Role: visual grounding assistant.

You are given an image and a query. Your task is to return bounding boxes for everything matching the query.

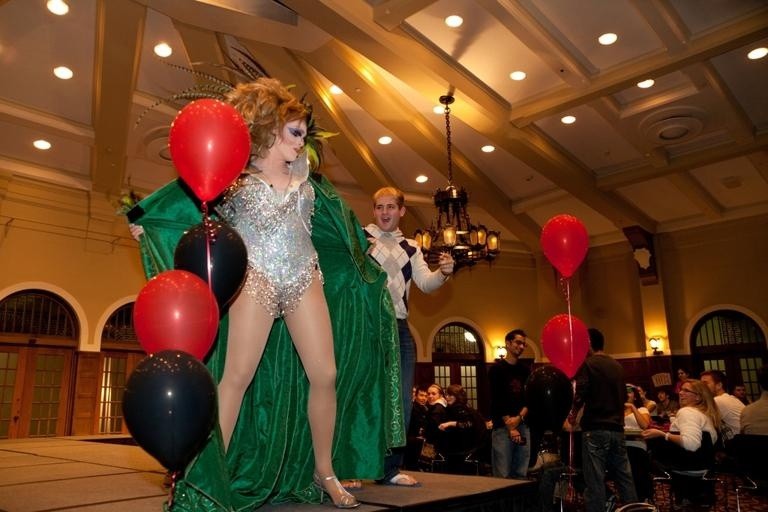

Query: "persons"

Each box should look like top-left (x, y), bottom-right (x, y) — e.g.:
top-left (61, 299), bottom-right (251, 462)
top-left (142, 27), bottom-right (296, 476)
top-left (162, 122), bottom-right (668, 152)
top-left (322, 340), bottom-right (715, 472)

top-left (358, 182), bottom-right (457, 487)
top-left (124, 75), bottom-right (409, 512)
top-left (412, 383), bottom-right (474, 471)
top-left (623, 367), bottom-right (768, 486)
top-left (567, 327), bottom-right (638, 511)
top-left (476, 327), bottom-right (534, 480)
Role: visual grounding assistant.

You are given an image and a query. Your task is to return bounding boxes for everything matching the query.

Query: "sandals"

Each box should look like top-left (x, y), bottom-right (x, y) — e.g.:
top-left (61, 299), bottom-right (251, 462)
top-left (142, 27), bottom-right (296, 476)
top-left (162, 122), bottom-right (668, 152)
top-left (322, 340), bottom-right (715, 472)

top-left (390, 473), bottom-right (421, 487)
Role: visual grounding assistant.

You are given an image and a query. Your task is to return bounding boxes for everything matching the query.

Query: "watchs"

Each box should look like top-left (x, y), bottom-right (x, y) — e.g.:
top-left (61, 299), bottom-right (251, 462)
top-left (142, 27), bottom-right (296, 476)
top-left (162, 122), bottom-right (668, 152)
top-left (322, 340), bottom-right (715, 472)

top-left (517, 412), bottom-right (526, 424)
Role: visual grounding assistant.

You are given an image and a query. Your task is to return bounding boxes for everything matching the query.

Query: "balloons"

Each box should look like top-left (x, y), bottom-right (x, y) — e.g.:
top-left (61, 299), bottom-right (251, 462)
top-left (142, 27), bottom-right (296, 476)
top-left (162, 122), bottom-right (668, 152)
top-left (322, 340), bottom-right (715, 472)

top-left (172, 219), bottom-right (248, 310)
top-left (120, 351), bottom-right (221, 476)
top-left (132, 268), bottom-right (222, 362)
top-left (540, 314), bottom-right (590, 382)
top-left (539, 213), bottom-right (591, 283)
top-left (168, 97), bottom-right (251, 202)
top-left (524, 368), bottom-right (573, 433)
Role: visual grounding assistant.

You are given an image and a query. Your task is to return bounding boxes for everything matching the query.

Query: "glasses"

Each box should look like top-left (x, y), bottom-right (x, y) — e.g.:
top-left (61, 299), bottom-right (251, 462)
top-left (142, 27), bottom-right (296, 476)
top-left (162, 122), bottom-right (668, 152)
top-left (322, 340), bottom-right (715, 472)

top-left (680, 387), bottom-right (698, 395)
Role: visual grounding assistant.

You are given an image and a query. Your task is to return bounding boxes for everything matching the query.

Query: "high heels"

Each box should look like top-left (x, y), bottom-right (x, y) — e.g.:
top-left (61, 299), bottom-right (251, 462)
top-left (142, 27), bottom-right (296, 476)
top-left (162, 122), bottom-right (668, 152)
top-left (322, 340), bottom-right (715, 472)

top-left (313, 472), bottom-right (361, 509)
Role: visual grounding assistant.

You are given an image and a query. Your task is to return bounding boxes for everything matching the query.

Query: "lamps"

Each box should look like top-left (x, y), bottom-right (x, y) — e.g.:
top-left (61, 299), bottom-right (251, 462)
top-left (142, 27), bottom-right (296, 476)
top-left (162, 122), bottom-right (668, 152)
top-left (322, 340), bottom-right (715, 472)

top-left (648, 337), bottom-right (664, 356)
top-left (496, 345), bottom-right (506, 358)
top-left (415, 93), bottom-right (500, 270)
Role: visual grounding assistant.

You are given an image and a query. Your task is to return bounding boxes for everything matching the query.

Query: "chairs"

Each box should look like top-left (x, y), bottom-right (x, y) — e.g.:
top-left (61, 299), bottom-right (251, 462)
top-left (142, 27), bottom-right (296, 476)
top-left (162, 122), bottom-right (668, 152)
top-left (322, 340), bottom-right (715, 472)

top-left (399, 430), bottom-right (767, 511)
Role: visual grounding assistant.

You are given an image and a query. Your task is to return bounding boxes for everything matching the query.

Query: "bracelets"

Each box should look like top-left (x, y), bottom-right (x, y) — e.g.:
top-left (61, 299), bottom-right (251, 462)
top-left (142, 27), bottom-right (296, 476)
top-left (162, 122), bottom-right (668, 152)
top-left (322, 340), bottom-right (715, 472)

top-left (569, 410), bottom-right (577, 418)
